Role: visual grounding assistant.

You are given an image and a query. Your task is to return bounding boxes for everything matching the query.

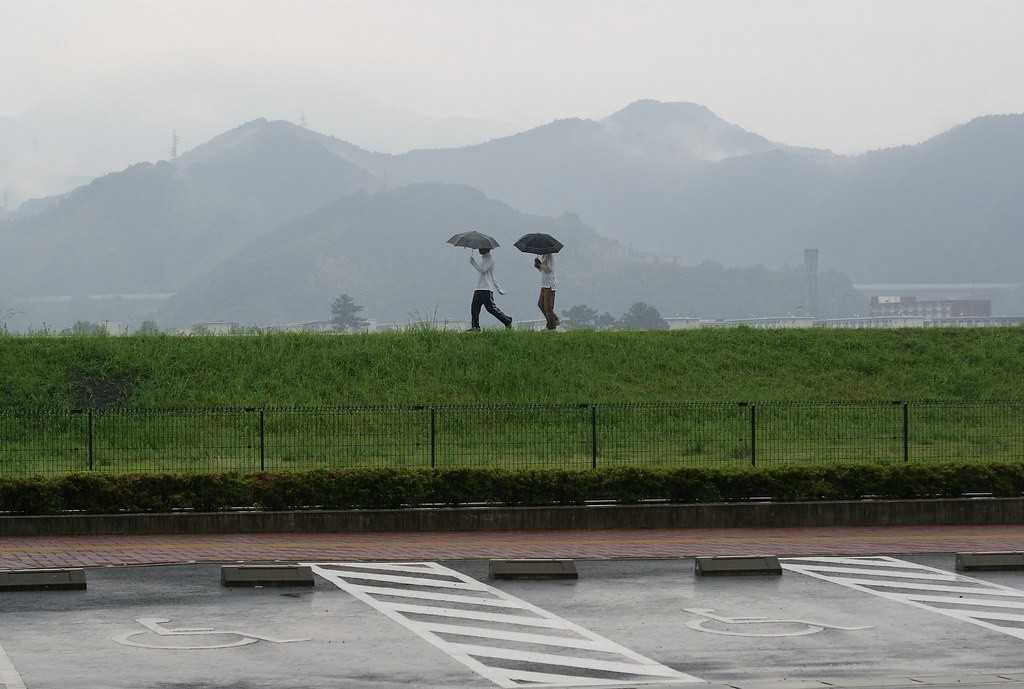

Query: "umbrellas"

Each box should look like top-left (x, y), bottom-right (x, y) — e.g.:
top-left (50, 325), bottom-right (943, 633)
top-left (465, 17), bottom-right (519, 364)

top-left (446, 231), bottom-right (500, 257)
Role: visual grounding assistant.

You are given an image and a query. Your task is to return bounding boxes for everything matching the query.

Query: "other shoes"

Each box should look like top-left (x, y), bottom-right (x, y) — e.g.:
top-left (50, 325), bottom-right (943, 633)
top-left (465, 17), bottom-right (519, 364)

top-left (466, 327), bottom-right (481, 332)
top-left (546, 318), bottom-right (561, 328)
top-left (506, 317), bottom-right (512, 330)
top-left (542, 328), bottom-right (557, 332)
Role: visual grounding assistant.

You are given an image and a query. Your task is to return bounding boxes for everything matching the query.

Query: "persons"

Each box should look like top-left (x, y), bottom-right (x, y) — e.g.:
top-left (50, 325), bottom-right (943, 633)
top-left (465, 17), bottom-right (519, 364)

top-left (534, 253), bottom-right (560, 331)
top-left (467, 248), bottom-right (513, 332)
top-left (513, 232), bottom-right (564, 256)
